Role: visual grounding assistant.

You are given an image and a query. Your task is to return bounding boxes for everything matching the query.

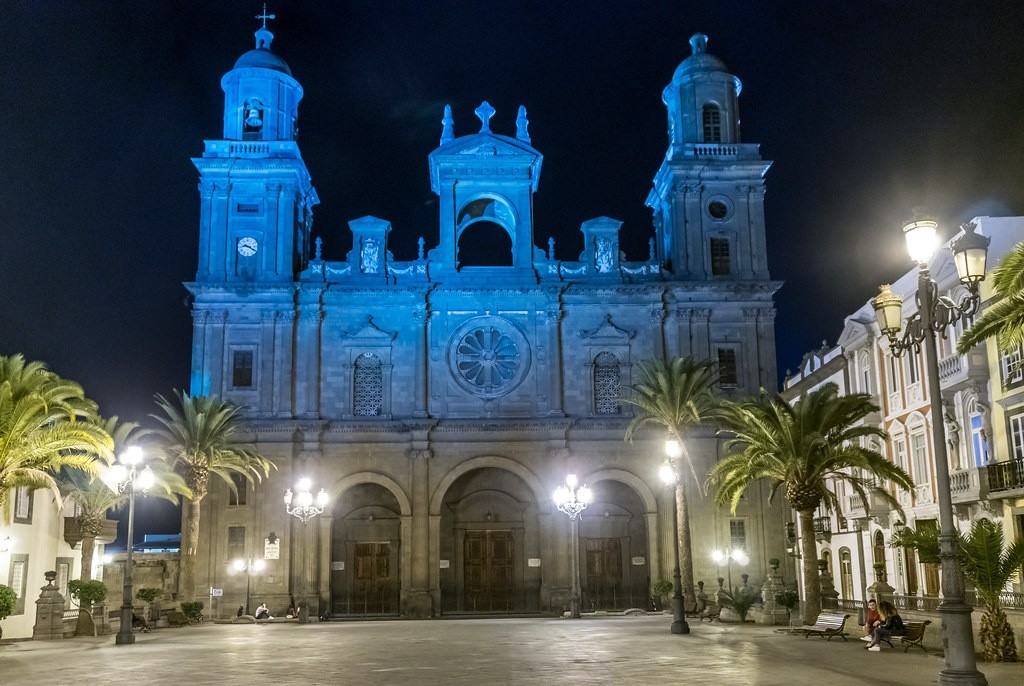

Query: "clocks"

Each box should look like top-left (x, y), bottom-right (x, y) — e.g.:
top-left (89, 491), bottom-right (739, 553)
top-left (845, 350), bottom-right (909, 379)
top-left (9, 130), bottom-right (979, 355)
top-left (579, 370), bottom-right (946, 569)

top-left (238, 237), bottom-right (258, 257)
top-left (787, 544), bottom-right (802, 559)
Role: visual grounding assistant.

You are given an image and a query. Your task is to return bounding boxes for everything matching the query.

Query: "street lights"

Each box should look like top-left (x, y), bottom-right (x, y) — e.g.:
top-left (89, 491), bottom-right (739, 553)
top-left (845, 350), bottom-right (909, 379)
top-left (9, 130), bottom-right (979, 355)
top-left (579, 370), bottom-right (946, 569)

top-left (283, 477), bottom-right (329, 621)
top-left (712, 548), bottom-right (750, 596)
top-left (872, 203), bottom-right (992, 686)
top-left (111, 446), bottom-right (156, 642)
top-left (553, 473), bottom-right (592, 618)
top-left (234, 557), bottom-right (266, 615)
top-left (658, 427), bottom-right (691, 633)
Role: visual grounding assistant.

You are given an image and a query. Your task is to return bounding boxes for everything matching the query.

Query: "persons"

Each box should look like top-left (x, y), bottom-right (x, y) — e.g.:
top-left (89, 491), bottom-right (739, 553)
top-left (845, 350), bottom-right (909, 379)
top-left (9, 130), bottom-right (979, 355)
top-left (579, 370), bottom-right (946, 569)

top-left (863, 599), bottom-right (881, 648)
top-left (976, 402), bottom-right (993, 462)
top-left (944, 414), bottom-right (961, 470)
top-left (295, 603), bottom-right (300, 615)
top-left (319, 608), bottom-right (330, 622)
top-left (255, 603), bottom-right (274, 619)
top-left (285, 603), bottom-right (296, 619)
top-left (860, 601), bottom-right (905, 651)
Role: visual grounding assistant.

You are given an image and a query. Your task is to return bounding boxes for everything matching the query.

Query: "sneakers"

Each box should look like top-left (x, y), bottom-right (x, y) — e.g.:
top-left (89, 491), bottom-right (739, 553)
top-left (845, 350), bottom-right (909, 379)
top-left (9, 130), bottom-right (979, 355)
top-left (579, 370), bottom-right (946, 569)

top-left (868, 646), bottom-right (880, 651)
top-left (859, 635), bottom-right (872, 642)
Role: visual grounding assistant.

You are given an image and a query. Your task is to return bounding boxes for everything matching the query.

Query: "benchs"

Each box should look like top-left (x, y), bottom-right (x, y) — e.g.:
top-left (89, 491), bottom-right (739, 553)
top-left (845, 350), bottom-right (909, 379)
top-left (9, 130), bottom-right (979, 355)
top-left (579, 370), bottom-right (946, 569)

top-left (166, 612), bottom-right (194, 628)
top-left (132, 611), bottom-right (152, 633)
top-left (801, 612), bottom-right (851, 641)
top-left (698, 604), bottom-right (723, 623)
top-left (684, 602), bottom-right (698, 618)
top-left (864, 619), bottom-right (931, 653)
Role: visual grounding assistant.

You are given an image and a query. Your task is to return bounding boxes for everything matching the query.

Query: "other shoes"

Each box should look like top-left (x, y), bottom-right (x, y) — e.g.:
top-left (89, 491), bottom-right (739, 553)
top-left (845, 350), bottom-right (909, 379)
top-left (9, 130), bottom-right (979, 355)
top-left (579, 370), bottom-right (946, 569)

top-left (864, 644), bottom-right (869, 649)
top-left (268, 616), bottom-right (275, 619)
